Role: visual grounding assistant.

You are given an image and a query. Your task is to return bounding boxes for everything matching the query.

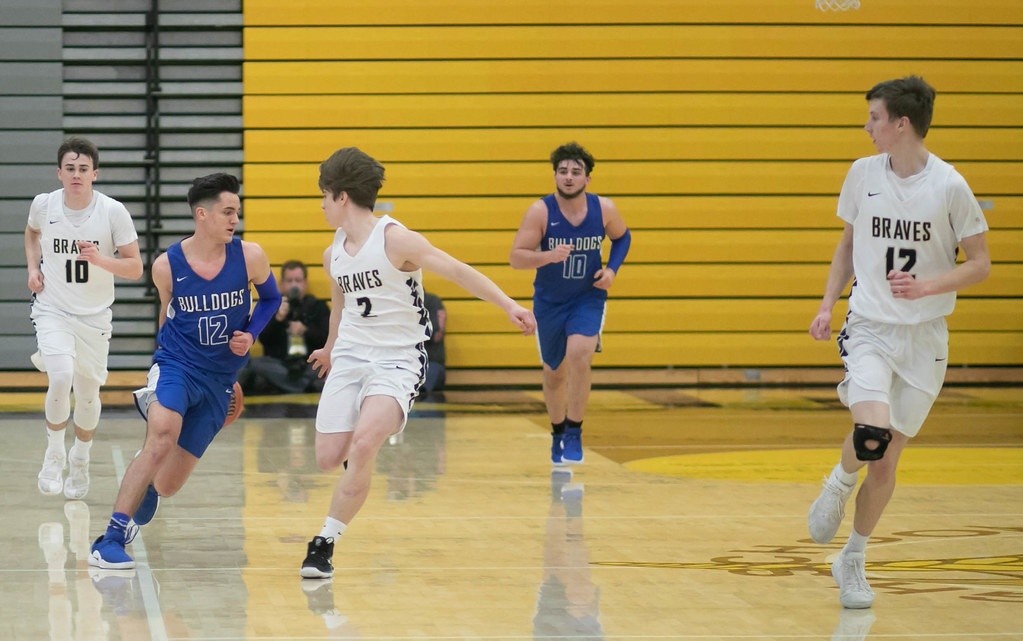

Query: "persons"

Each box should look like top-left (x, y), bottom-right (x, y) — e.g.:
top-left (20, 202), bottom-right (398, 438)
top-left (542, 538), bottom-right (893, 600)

top-left (25, 139), bottom-right (143, 500)
top-left (236, 261), bottom-right (331, 395)
top-left (87, 174), bottom-right (282, 569)
top-left (510, 141), bottom-right (631, 466)
top-left (805, 76), bottom-right (990, 609)
top-left (416, 287), bottom-right (448, 404)
top-left (299, 148), bottom-right (537, 578)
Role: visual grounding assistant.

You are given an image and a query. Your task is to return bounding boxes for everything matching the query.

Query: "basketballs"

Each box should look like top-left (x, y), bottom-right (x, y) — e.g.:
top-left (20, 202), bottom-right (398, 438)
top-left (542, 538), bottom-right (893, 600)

top-left (223, 381), bottom-right (244, 428)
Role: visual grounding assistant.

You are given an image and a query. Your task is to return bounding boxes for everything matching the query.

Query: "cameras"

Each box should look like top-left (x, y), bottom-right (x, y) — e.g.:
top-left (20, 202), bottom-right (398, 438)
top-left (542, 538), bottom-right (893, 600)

top-left (283, 287), bottom-right (303, 321)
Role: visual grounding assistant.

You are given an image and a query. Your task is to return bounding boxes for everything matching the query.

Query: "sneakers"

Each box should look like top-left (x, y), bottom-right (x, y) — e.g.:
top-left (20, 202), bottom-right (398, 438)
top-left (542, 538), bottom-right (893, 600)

top-left (38, 451), bottom-right (67, 495)
top-left (39, 522), bottom-right (68, 564)
top-left (832, 608), bottom-right (877, 641)
top-left (808, 467), bottom-right (857, 544)
top-left (64, 500), bottom-right (90, 553)
top-left (64, 446), bottom-right (90, 499)
top-left (831, 544), bottom-right (875, 609)
top-left (87, 523), bottom-right (140, 569)
top-left (87, 566), bottom-right (137, 616)
top-left (300, 576), bottom-right (335, 615)
top-left (551, 468), bottom-right (573, 503)
top-left (561, 483), bottom-right (584, 514)
top-left (560, 427), bottom-right (584, 463)
top-left (344, 459), bottom-right (348, 470)
top-left (550, 431), bottom-right (570, 466)
top-left (131, 571), bottom-right (160, 612)
top-left (133, 450), bottom-right (161, 525)
top-left (301, 536), bottom-right (334, 577)
